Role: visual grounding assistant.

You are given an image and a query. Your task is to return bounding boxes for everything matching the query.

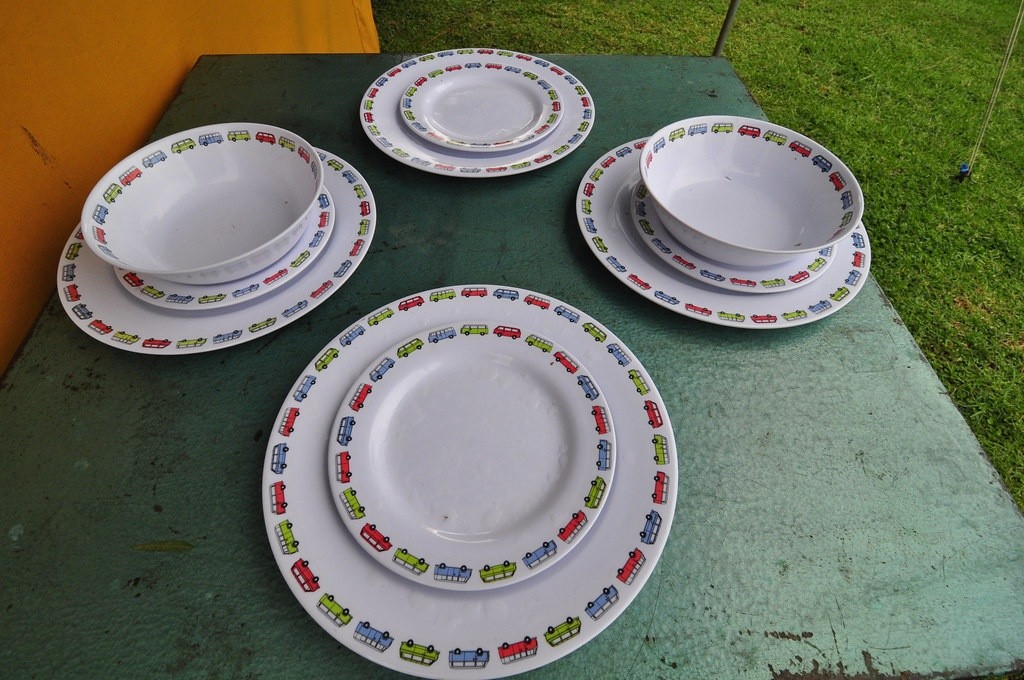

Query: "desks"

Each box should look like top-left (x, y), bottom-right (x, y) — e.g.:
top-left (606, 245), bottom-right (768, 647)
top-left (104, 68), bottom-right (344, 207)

top-left (0, 50), bottom-right (1024, 680)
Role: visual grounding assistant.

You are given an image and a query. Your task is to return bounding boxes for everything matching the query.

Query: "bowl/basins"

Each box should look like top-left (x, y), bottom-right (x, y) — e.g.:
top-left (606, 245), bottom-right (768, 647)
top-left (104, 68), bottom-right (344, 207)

top-left (640, 114), bottom-right (864, 266)
top-left (82, 123), bottom-right (323, 286)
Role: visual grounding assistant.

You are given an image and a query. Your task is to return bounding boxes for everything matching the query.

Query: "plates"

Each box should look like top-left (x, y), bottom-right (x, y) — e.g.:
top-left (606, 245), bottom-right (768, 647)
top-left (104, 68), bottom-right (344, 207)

top-left (57, 147), bottom-right (377, 355)
top-left (631, 179), bottom-right (836, 293)
top-left (575, 136), bottom-right (871, 330)
top-left (326, 320), bottom-right (617, 591)
top-left (261, 284), bottom-right (679, 680)
top-left (112, 186), bottom-right (336, 310)
top-left (360, 48), bottom-right (595, 178)
top-left (399, 62), bottom-right (564, 153)
